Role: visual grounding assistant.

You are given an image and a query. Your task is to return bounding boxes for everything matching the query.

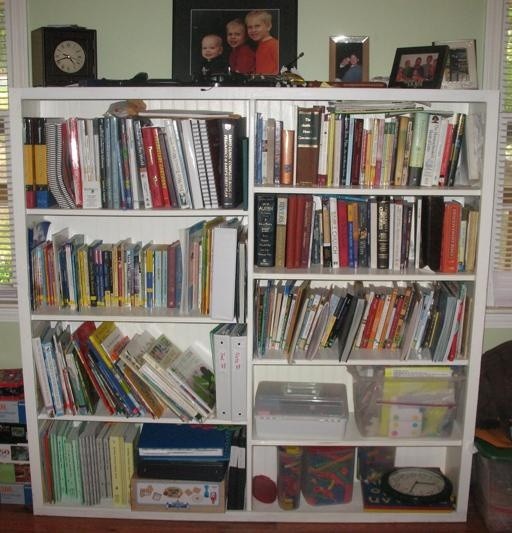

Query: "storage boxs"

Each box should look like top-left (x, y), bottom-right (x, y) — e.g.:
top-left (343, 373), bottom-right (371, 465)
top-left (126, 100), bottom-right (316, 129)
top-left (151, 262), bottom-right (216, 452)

top-left (351, 376), bottom-right (466, 439)
top-left (474, 427), bottom-right (512, 533)
top-left (255, 381), bottom-right (349, 441)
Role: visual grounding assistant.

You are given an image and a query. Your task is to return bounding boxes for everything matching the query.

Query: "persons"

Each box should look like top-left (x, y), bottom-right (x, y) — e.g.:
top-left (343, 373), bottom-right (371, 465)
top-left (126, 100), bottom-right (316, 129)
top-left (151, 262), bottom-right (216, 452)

top-left (189, 9), bottom-right (445, 88)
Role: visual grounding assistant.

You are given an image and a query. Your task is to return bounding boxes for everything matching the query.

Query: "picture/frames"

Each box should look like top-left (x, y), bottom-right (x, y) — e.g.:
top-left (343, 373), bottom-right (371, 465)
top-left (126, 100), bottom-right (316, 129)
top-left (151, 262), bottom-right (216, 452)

top-left (388, 45), bottom-right (449, 88)
top-left (329, 37), bottom-right (370, 83)
top-left (172, 1), bottom-right (298, 87)
top-left (434, 39), bottom-right (479, 89)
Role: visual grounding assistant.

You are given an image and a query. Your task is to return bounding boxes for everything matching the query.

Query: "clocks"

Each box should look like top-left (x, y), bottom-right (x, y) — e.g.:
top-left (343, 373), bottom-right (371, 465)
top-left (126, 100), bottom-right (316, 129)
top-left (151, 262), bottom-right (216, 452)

top-left (31, 24), bottom-right (97, 87)
top-left (384, 467), bottom-right (453, 504)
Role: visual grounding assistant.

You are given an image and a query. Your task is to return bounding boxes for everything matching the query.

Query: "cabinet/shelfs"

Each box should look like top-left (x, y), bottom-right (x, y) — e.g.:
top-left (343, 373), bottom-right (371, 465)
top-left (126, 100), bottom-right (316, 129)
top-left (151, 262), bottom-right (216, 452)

top-left (8, 87), bottom-right (503, 523)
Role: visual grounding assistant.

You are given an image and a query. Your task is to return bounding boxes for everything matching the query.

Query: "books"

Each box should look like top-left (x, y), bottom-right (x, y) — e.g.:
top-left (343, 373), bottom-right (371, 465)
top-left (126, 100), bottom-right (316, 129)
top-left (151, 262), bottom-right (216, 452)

top-left (255, 101), bottom-right (481, 188)
top-left (31, 320), bottom-right (216, 423)
top-left (27, 217), bottom-right (246, 324)
top-left (255, 280), bottom-right (473, 363)
top-left (136, 422), bottom-right (229, 481)
top-left (24, 109), bottom-right (246, 210)
top-left (227, 445), bottom-right (245, 510)
top-left (256, 193), bottom-right (480, 273)
top-left (38, 421), bottom-right (140, 508)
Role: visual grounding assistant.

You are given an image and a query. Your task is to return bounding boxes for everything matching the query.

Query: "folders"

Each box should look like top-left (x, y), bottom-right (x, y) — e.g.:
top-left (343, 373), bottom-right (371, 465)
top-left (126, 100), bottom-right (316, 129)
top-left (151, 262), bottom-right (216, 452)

top-left (213, 326), bottom-right (247, 423)
